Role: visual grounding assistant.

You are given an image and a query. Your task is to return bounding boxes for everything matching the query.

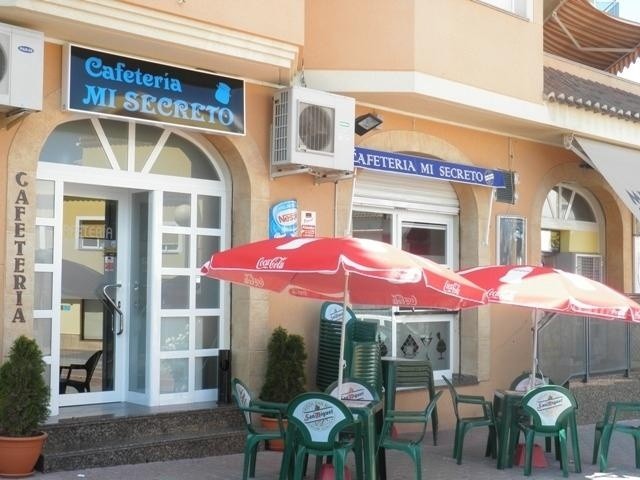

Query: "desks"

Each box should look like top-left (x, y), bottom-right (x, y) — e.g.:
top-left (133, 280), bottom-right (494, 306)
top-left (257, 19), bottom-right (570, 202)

top-left (300, 395), bottom-right (389, 480)
top-left (482, 385), bottom-right (567, 471)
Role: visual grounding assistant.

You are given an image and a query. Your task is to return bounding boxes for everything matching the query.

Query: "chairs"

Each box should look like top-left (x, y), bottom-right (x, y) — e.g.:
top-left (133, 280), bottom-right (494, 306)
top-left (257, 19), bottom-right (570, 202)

top-left (326, 370), bottom-right (381, 410)
top-left (275, 391), bottom-right (363, 478)
top-left (374, 390), bottom-right (448, 480)
top-left (59, 349), bottom-right (108, 397)
top-left (312, 297), bottom-right (444, 445)
top-left (435, 368), bottom-right (505, 467)
top-left (507, 384), bottom-right (587, 480)
top-left (507, 369), bottom-right (560, 451)
top-left (589, 395), bottom-right (640, 474)
top-left (231, 372), bottom-right (298, 479)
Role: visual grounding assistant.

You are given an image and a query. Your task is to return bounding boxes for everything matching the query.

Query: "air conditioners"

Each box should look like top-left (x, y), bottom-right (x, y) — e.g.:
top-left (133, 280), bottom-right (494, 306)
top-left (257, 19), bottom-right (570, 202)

top-left (0, 21), bottom-right (46, 116)
top-left (270, 82), bottom-right (359, 182)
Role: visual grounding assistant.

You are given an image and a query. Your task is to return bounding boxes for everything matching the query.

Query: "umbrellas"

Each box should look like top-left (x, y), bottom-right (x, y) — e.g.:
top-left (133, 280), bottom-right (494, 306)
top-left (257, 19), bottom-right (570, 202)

top-left (450, 263), bottom-right (640, 393)
top-left (197, 235), bottom-right (491, 405)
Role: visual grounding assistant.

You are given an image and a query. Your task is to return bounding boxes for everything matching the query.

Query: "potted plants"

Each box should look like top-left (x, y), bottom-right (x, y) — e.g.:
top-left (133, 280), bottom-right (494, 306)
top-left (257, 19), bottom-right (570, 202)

top-left (254, 321), bottom-right (310, 452)
top-left (0, 333), bottom-right (52, 477)
